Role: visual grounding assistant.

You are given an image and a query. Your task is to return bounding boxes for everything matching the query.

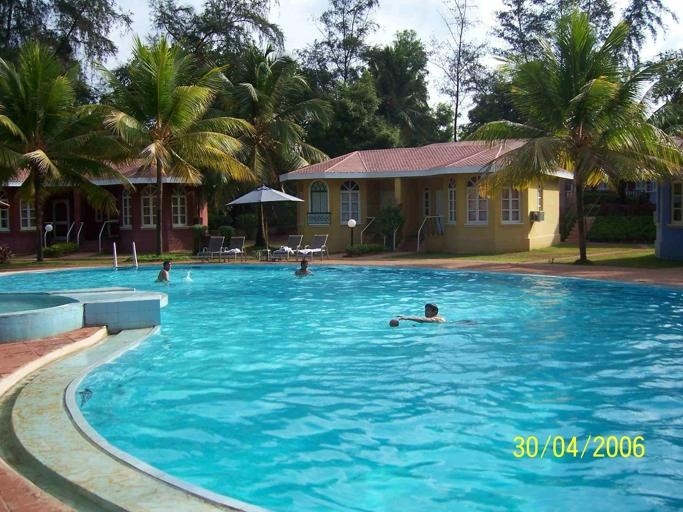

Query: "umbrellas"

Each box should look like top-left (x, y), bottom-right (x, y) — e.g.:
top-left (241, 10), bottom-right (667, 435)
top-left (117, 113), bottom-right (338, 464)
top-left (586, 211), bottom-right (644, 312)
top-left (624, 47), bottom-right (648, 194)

top-left (225, 183), bottom-right (305, 250)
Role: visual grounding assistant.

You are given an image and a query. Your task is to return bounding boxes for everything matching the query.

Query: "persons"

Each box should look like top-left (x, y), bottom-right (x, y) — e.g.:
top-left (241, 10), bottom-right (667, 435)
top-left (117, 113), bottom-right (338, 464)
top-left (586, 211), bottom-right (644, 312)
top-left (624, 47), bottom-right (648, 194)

top-left (293, 259), bottom-right (313, 276)
top-left (154, 260), bottom-right (172, 283)
top-left (395, 302), bottom-right (446, 324)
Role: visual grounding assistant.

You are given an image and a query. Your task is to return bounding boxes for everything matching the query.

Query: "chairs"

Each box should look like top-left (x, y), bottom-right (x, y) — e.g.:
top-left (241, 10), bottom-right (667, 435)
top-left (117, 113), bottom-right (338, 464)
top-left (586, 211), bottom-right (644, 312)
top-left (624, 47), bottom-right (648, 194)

top-left (195, 234), bottom-right (330, 262)
